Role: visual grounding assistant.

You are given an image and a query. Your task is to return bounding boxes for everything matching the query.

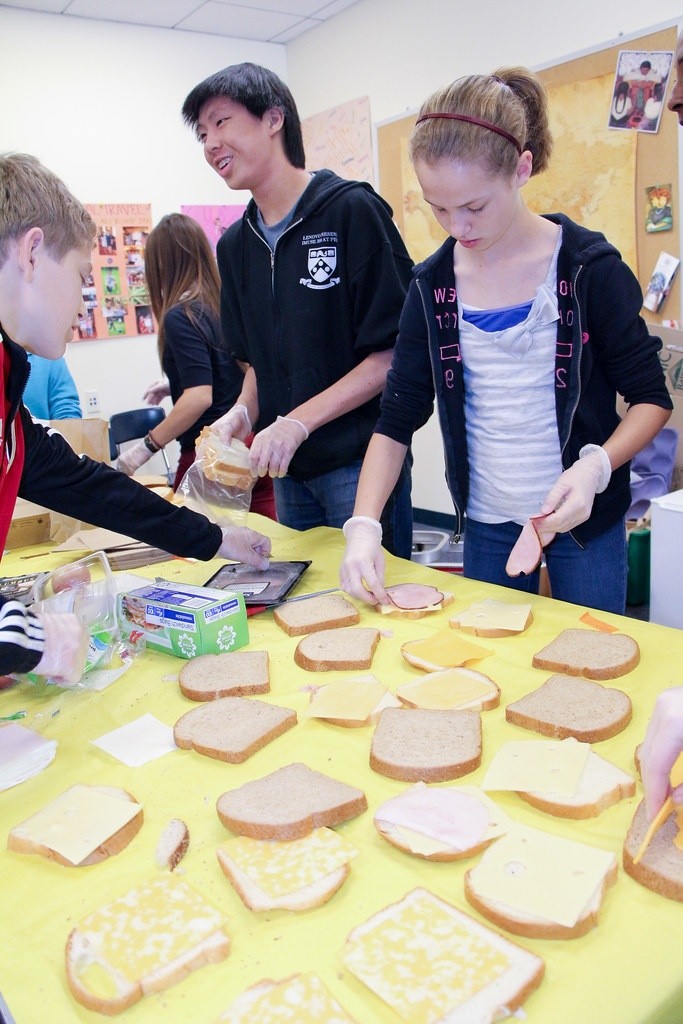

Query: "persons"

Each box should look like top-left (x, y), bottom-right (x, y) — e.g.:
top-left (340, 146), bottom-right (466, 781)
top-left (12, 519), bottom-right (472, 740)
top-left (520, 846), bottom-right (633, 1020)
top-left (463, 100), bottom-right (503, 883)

top-left (180, 62), bottom-right (416, 560)
top-left (21, 350), bottom-right (82, 420)
top-left (0, 150), bottom-right (271, 688)
top-left (115, 214), bottom-right (275, 521)
top-left (636, 30), bottom-right (683, 827)
top-left (341, 67), bottom-right (674, 616)
top-left (77, 227), bottom-right (154, 337)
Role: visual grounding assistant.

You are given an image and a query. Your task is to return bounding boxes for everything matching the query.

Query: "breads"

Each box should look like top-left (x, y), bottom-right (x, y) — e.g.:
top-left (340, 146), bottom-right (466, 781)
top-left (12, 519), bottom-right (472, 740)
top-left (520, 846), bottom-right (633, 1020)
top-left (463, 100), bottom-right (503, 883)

top-left (174, 697), bottom-right (298, 763)
top-left (274, 594), bottom-right (360, 636)
top-left (373, 786), bottom-right (497, 861)
top-left (8, 784), bottom-right (142, 867)
top-left (347, 891), bottom-right (546, 1024)
top-left (622, 785), bottom-right (683, 902)
top-left (533, 628), bottom-right (641, 679)
top-left (374, 588), bottom-right (452, 619)
top-left (219, 974), bottom-right (358, 1024)
top-left (154, 818), bottom-right (190, 871)
top-left (396, 666), bottom-right (500, 713)
top-left (294, 627), bottom-right (380, 671)
top-left (510, 738), bottom-right (637, 819)
top-left (402, 640), bottom-right (465, 671)
top-left (466, 824), bottom-right (618, 940)
top-left (194, 426), bottom-right (254, 489)
top-left (150, 486), bottom-right (174, 501)
top-left (506, 672), bottom-right (633, 743)
top-left (66, 884), bottom-right (231, 1015)
top-left (180, 652), bottom-right (269, 701)
top-left (311, 675), bottom-right (400, 729)
top-left (450, 599), bottom-right (532, 637)
top-left (216, 827), bottom-right (350, 914)
top-left (370, 707), bottom-right (483, 782)
top-left (217, 765), bottom-right (367, 841)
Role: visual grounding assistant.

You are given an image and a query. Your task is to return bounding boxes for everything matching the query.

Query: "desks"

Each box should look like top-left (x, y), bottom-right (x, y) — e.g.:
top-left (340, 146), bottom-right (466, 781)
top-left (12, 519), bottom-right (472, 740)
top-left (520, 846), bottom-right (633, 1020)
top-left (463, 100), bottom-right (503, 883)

top-left (1, 495), bottom-right (683, 1024)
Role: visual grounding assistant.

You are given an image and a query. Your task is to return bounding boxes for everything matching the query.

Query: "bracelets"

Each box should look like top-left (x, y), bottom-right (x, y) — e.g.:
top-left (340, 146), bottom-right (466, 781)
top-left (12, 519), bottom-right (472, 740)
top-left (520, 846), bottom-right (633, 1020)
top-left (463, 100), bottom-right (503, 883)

top-left (148, 430), bottom-right (165, 449)
top-left (144, 434), bottom-right (159, 453)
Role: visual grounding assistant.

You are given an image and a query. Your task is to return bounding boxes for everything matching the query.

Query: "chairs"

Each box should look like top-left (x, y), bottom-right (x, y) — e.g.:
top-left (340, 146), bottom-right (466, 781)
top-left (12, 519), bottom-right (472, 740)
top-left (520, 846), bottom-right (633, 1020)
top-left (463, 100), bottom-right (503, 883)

top-left (110, 407), bottom-right (174, 487)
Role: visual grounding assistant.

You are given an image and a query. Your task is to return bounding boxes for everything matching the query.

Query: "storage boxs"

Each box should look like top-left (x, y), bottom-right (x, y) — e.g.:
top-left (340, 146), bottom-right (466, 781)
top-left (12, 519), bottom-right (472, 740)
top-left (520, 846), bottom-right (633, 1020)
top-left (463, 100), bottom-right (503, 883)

top-left (116, 576), bottom-right (250, 661)
